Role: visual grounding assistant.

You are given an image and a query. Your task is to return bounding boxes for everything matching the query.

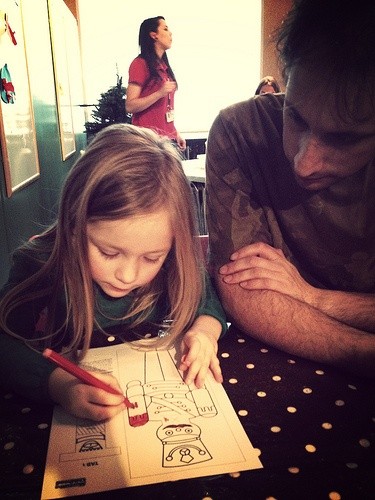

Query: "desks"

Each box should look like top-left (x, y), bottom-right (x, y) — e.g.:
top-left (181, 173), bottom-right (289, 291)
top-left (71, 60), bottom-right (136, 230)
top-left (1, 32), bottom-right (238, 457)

top-left (0, 319), bottom-right (375, 500)
top-left (183, 154), bottom-right (210, 261)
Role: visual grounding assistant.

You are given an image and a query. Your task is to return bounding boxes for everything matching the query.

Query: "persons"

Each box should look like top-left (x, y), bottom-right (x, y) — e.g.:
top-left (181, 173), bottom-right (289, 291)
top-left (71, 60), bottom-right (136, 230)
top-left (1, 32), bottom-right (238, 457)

top-left (255, 77), bottom-right (282, 99)
top-left (0, 123), bottom-right (226, 421)
top-left (206, 0), bottom-right (375, 362)
top-left (125, 17), bottom-right (186, 153)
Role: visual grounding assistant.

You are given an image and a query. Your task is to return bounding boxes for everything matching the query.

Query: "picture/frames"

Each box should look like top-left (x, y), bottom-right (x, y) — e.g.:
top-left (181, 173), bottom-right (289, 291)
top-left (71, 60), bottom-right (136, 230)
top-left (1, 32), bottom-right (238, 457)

top-left (0, 0), bottom-right (43, 201)
top-left (46, 0), bottom-right (77, 162)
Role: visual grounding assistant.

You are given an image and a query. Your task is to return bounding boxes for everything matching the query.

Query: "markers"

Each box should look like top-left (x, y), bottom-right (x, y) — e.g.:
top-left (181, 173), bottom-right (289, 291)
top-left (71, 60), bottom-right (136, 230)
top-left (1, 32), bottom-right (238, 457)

top-left (43, 347), bottom-right (136, 410)
